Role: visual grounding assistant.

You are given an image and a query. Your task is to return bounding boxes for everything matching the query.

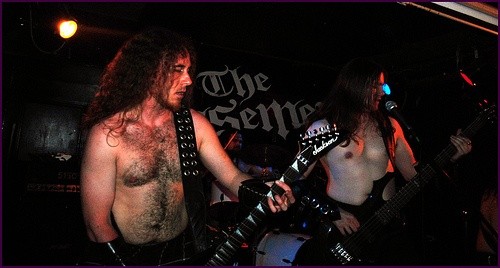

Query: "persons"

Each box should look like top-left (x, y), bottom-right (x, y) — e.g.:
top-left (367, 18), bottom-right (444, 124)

top-left (210, 128), bottom-right (275, 202)
top-left (288, 56), bottom-right (473, 237)
top-left (75, 30), bottom-right (295, 267)
top-left (475, 165), bottom-right (500, 268)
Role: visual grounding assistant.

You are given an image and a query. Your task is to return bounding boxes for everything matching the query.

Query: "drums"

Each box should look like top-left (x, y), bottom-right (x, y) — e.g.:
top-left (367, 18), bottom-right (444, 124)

top-left (253, 227), bottom-right (313, 267)
top-left (207, 176), bottom-right (249, 228)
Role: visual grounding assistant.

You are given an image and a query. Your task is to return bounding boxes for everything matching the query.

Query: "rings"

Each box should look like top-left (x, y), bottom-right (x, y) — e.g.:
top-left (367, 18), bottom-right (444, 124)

top-left (467, 142), bottom-right (471, 145)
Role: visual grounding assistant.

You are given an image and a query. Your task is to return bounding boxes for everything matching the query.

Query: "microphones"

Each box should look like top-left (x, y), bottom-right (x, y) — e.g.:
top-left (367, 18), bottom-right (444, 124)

top-left (385, 100), bottom-right (410, 130)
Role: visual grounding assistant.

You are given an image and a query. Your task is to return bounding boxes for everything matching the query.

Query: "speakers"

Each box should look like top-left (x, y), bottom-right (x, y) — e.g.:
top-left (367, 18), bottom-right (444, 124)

top-left (476, 189), bottom-right (497, 255)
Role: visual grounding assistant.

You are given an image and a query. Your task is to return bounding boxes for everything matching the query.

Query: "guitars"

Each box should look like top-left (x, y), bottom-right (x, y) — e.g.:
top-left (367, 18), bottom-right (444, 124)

top-left (204, 115), bottom-right (344, 266)
top-left (291, 94), bottom-right (499, 267)
